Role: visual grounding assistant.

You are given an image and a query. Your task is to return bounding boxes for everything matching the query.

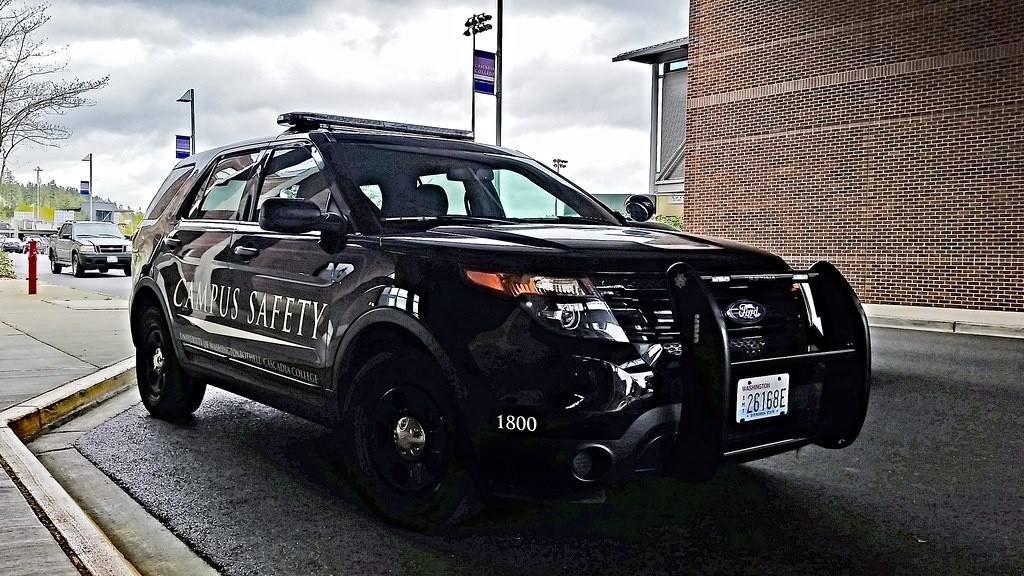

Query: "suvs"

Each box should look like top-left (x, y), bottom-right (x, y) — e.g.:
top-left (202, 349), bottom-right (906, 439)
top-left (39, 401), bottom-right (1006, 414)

top-left (48, 220), bottom-right (133, 278)
top-left (120, 111), bottom-right (874, 534)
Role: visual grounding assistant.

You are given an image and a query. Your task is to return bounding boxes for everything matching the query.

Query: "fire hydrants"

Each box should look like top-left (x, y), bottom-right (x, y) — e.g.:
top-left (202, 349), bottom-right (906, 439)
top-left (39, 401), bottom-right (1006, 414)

top-left (23, 238), bottom-right (39, 296)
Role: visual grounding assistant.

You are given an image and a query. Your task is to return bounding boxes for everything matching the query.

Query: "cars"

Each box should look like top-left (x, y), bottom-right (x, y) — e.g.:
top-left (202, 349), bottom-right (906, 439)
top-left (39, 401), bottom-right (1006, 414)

top-left (2, 237), bottom-right (22, 254)
top-left (19, 235), bottom-right (47, 254)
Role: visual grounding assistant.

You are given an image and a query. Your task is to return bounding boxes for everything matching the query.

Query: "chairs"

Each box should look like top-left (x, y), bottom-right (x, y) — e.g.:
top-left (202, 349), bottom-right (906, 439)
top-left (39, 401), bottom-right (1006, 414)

top-left (293, 168), bottom-right (365, 213)
top-left (406, 183), bottom-right (450, 218)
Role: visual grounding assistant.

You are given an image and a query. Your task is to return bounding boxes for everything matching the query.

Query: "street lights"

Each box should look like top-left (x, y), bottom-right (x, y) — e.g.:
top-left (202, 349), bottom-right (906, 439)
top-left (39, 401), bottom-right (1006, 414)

top-left (36, 169), bottom-right (45, 219)
top-left (552, 159), bottom-right (569, 215)
top-left (462, 12), bottom-right (493, 140)
top-left (81, 152), bottom-right (92, 221)
top-left (175, 88), bottom-right (196, 155)
